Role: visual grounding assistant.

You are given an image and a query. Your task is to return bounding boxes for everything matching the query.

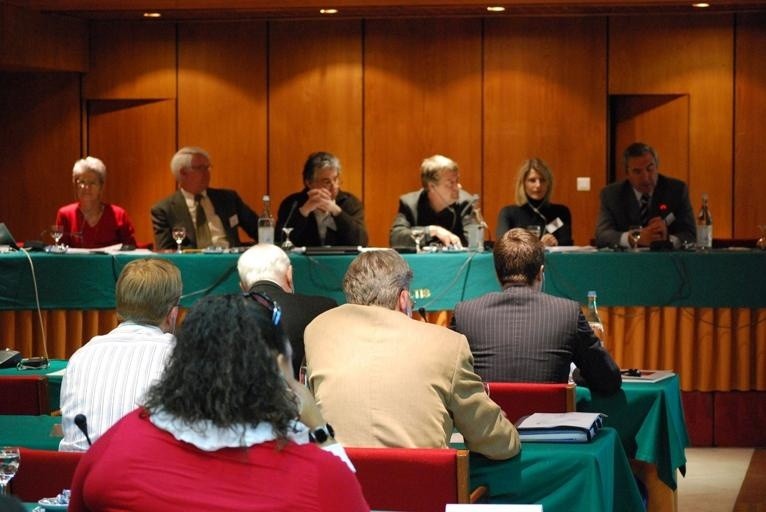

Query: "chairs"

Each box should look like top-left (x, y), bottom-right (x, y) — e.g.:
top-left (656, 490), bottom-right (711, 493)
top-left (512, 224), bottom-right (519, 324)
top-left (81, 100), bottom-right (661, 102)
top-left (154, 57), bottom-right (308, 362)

top-left (5, 447), bottom-right (86, 502)
top-left (0, 376), bottom-right (50, 415)
top-left (481, 383), bottom-right (576, 425)
top-left (344, 447), bottom-right (469, 512)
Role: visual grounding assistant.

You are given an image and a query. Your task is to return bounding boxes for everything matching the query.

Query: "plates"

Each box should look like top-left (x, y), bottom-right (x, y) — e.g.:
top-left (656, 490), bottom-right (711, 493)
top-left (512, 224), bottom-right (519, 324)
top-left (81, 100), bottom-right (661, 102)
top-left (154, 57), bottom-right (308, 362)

top-left (37, 497), bottom-right (70, 511)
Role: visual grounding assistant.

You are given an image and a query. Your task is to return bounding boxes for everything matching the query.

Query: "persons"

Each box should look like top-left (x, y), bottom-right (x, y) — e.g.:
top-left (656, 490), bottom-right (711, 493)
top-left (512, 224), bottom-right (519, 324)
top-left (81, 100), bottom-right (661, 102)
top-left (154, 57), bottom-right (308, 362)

top-left (57, 256), bottom-right (184, 454)
top-left (448, 226), bottom-right (623, 398)
top-left (495, 157), bottom-right (574, 247)
top-left (69, 290), bottom-right (372, 512)
top-left (303, 248), bottom-right (522, 463)
top-left (593, 142), bottom-right (696, 252)
top-left (56, 154), bottom-right (139, 249)
top-left (237, 242), bottom-right (340, 383)
top-left (275, 151), bottom-right (369, 248)
top-left (389, 153), bottom-right (476, 253)
top-left (150, 145), bottom-right (260, 251)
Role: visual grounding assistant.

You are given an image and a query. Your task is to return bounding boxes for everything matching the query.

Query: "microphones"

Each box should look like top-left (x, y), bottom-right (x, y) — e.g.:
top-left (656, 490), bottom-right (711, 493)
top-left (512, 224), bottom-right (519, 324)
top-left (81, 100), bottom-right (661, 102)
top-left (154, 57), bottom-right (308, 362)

top-left (651, 188), bottom-right (673, 251)
top-left (75, 413), bottom-right (93, 446)
top-left (262, 195), bottom-right (273, 218)
top-left (418, 306), bottom-right (427, 322)
top-left (461, 193), bottom-right (481, 218)
top-left (278, 201), bottom-right (298, 247)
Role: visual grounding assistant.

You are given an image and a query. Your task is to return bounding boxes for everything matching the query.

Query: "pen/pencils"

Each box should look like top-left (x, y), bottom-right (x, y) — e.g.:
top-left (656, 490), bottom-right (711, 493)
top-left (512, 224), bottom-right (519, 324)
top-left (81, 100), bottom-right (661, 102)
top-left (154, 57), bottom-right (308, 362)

top-left (184, 249), bottom-right (204, 253)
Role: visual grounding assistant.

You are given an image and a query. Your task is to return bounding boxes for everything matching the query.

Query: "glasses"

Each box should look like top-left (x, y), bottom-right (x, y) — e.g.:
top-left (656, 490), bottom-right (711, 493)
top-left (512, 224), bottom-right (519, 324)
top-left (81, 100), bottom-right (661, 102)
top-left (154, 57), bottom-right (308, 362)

top-left (242, 290), bottom-right (283, 326)
top-left (76, 179), bottom-right (103, 188)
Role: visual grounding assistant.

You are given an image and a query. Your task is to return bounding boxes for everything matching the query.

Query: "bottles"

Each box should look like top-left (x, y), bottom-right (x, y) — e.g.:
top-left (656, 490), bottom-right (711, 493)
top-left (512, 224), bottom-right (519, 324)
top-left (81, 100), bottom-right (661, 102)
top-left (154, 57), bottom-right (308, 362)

top-left (468, 198), bottom-right (485, 255)
top-left (585, 289), bottom-right (605, 348)
top-left (697, 192), bottom-right (713, 252)
top-left (256, 193), bottom-right (276, 247)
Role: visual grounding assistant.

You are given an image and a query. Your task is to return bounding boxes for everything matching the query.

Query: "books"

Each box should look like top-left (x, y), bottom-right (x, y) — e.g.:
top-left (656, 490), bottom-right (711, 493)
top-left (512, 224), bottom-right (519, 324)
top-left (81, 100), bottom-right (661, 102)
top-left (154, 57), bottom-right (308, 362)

top-left (514, 414), bottom-right (605, 443)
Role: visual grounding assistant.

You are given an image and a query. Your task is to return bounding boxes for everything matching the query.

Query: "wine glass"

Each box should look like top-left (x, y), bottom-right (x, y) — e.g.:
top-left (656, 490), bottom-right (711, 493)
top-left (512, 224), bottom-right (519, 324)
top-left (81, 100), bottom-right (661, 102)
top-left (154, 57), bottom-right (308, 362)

top-left (0, 446), bottom-right (20, 495)
top-left (627, 225), bottom-right (643, 253)
top-left (410, 225), bottom-right (426, 254)
top-left (48, 225), bottom-right (63, 255)
top-left (172, 226), bottom-right (186, 254)
top-left (280, 227), bottom-right (296, 253)
top-left (754, 224), bottom-right (766, 251)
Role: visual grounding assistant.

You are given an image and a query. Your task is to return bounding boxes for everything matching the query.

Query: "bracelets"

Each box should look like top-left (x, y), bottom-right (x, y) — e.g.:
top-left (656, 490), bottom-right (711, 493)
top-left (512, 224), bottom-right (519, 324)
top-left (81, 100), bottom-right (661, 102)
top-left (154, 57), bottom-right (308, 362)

top-left (308, 422), bottom-right (335, 444)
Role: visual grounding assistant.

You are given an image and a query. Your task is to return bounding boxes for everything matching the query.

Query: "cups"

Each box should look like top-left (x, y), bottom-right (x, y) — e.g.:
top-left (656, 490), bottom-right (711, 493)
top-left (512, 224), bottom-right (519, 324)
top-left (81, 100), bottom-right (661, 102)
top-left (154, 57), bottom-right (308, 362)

top-left (528, 225), bottom-right (541, 238)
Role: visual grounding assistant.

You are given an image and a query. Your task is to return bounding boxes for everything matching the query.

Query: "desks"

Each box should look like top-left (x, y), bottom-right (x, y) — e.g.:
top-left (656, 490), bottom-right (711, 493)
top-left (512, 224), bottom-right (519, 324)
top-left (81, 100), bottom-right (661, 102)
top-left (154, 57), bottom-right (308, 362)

top-left (0, 358), bottom-right (688, 512)
top-left (0, 415), bottom-right (648, 512)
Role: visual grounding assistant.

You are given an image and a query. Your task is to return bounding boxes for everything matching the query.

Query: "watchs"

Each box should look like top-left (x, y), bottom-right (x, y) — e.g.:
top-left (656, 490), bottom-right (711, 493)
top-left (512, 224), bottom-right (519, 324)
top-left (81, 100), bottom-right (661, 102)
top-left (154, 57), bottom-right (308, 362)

top-left (631, 230), bottom-right (640, 243)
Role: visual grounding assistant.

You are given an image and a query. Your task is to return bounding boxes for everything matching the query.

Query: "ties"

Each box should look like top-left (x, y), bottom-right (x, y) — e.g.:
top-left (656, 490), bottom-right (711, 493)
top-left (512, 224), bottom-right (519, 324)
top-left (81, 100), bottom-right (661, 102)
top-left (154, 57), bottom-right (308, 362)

top-left (194, 193), bottom-right (213, 249)
top-left (190, 164), bottom-right (214, 172)
top-left (640, 192), bottom-right (652, 228)
